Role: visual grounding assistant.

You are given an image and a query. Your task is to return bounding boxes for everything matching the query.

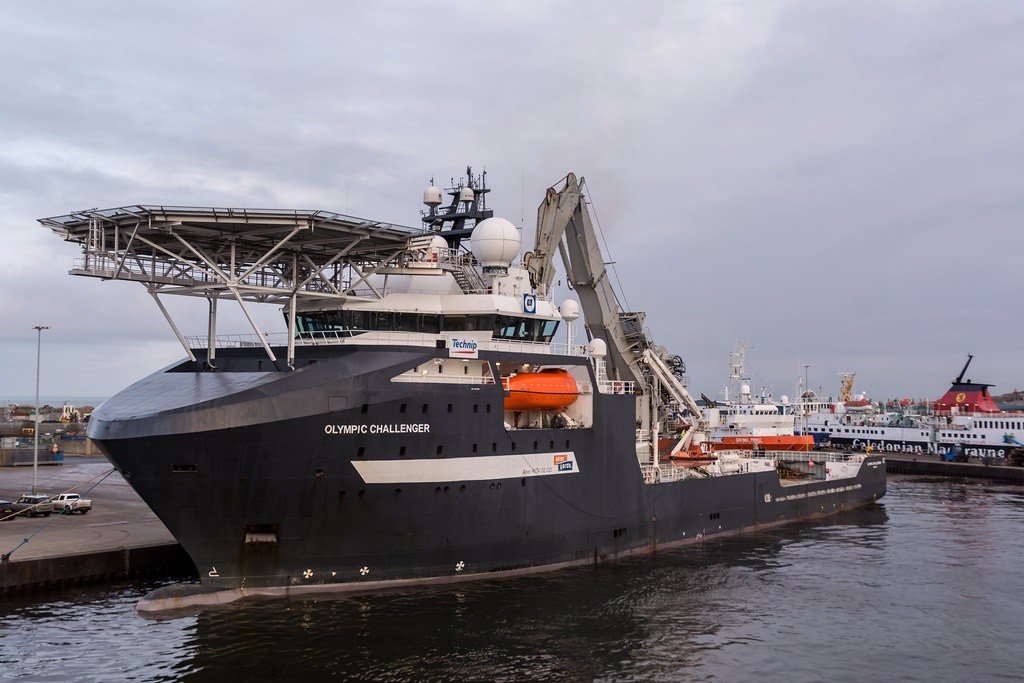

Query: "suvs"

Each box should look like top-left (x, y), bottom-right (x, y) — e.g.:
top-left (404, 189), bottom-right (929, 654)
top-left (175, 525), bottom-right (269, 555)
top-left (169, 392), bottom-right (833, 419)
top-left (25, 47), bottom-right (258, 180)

top-left (12, 493), bottom-right (54, 518)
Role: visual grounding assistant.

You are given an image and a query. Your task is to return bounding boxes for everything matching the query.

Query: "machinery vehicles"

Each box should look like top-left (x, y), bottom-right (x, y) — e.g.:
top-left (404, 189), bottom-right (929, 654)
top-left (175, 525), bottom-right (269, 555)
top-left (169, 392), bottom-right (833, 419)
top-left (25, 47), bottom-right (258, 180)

top-left (941, 445), bottom-right (972, 462)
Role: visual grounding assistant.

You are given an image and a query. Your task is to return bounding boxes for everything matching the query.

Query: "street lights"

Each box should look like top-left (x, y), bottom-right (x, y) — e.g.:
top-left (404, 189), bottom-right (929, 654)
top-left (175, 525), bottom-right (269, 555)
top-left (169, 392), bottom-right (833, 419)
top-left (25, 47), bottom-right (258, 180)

top-left (804, 366), bottom-right (810, 434)
top-left (32, 325), bottom-right (49, 495)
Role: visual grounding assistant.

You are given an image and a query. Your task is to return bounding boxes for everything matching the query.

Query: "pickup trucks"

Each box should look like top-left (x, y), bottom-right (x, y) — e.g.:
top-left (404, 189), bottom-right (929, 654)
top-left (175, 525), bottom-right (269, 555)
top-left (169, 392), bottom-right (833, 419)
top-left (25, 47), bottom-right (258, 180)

top-left (51, 493), bottom-right (92, 515)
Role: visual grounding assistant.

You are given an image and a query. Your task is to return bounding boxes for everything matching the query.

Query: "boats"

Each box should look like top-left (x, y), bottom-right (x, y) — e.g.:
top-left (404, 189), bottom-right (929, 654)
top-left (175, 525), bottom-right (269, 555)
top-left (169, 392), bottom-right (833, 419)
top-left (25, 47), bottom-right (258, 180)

top-left (36, 158), bottom-right (888, 619)
top-left (658, 339), bottom-right (1024, 455)
top-left (667, 444), bottom-right (719, 467)
top-left (501, 367), bottom-right (579, 411)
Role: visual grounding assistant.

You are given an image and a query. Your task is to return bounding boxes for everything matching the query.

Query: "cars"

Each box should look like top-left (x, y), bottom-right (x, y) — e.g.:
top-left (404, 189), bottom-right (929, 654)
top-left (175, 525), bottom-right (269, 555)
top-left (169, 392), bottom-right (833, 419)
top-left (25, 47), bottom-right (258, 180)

top-left (0, 500), bottom-right (15, 520)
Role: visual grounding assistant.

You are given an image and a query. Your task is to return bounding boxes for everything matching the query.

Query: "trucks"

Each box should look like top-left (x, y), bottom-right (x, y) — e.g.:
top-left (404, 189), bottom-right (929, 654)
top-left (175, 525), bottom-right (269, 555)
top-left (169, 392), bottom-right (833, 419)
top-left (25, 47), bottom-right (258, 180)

top-left (795, 431), bottom-right (831, 450)
